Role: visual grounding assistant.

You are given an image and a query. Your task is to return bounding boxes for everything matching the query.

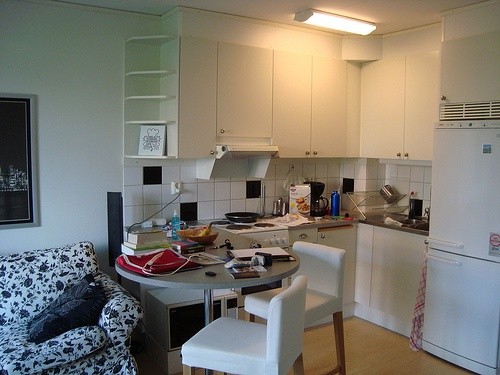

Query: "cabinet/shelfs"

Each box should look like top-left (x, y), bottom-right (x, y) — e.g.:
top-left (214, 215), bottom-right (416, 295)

top-left (239, 225), bottom-right (429, 319)
top-left (122, 7), bottom-right (443, 159)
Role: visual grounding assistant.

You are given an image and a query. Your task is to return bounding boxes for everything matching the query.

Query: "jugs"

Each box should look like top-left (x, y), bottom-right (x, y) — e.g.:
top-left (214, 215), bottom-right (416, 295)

top-left (313, 196), bottom-right (328, 211)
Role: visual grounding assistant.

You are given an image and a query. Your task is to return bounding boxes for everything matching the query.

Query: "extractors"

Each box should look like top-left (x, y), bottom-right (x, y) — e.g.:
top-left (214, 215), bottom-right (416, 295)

top-left (215, 143), bottom-right (278, 159)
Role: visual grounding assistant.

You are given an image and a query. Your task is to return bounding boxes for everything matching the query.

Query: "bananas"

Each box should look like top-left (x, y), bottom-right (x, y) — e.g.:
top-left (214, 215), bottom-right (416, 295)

top-left (195, 222), bottom-right (212, 236)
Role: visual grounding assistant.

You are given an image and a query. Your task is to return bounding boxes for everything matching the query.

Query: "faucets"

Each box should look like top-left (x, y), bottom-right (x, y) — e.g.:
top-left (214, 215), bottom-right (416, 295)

top-left (423, 207), bottom-right (430, 222)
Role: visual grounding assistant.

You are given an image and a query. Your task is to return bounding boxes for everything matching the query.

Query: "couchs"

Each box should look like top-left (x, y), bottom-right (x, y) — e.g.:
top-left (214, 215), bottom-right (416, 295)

top-left (0, 240), bottom-right (145, 375)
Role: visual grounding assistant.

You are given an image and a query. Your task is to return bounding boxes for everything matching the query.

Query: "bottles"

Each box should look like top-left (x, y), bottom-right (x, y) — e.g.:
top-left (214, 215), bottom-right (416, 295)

top-left (331, 190), bottom-right (340, 216)
top-left (273, 197), bottom-right (289, 216)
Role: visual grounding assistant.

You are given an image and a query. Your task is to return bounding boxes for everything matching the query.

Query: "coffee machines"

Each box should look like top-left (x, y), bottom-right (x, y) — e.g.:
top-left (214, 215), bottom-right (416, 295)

top-left (304, 181), bottom-right (329, 217)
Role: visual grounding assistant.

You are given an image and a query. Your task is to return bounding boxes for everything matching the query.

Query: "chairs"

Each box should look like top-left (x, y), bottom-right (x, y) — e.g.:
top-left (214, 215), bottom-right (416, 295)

top-left (181, 275), bottom-right (307, 375)
top-left (244, 241), bottom-right (347, 375)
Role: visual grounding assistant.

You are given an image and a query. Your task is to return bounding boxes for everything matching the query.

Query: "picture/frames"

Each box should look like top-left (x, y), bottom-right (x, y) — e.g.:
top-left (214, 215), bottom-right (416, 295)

top-left (0, 91), bottom-right (41, 229)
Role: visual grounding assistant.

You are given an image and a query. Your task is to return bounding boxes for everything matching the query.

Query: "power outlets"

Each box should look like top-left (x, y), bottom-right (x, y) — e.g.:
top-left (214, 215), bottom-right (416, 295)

top-left (171, 181), bottom-right (184, 195)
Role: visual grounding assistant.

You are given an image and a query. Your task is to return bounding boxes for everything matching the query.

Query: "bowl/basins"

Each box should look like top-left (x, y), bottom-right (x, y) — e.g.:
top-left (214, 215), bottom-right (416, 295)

top-left (176, 228), bottom-right (218, 245)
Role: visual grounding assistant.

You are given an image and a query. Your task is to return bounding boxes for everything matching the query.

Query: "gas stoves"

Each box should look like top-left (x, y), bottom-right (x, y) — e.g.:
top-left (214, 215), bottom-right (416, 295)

top-left (207, 220), bottom-right (289, 248)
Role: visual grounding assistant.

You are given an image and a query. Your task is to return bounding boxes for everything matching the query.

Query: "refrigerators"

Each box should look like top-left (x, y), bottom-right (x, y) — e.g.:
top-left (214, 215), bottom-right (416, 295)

top-left (421, 118), bottom-right (499, 375)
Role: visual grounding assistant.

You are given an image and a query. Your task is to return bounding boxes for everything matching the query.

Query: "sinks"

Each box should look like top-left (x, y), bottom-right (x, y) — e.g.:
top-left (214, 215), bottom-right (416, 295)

top-left (408, 223), bottom-right (429, 232)
top-left (382, 218), bottom-right (426, 227)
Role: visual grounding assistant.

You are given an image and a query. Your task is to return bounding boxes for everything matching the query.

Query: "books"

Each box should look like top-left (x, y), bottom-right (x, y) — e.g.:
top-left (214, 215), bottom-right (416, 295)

top-left (171, 238), bottom-right (205, 254)
top-left (229, 265), bottom-right (267, 279)
top-left (122, 229), bottom-right (172, 257)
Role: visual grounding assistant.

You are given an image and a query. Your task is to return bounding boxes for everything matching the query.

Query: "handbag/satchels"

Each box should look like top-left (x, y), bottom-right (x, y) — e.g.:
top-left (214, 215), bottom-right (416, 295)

top-left (117, 248), bottom-right (192, 275)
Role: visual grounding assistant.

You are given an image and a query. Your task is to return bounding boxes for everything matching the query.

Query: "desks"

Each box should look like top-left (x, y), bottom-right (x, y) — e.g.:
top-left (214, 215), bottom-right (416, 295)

top-left (114, 245), bottom-right (300, 375)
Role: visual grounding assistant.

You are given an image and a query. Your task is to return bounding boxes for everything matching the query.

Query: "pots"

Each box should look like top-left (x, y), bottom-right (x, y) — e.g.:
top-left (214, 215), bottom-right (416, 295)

top-left (225, 212), bottom-right (259, 223)
